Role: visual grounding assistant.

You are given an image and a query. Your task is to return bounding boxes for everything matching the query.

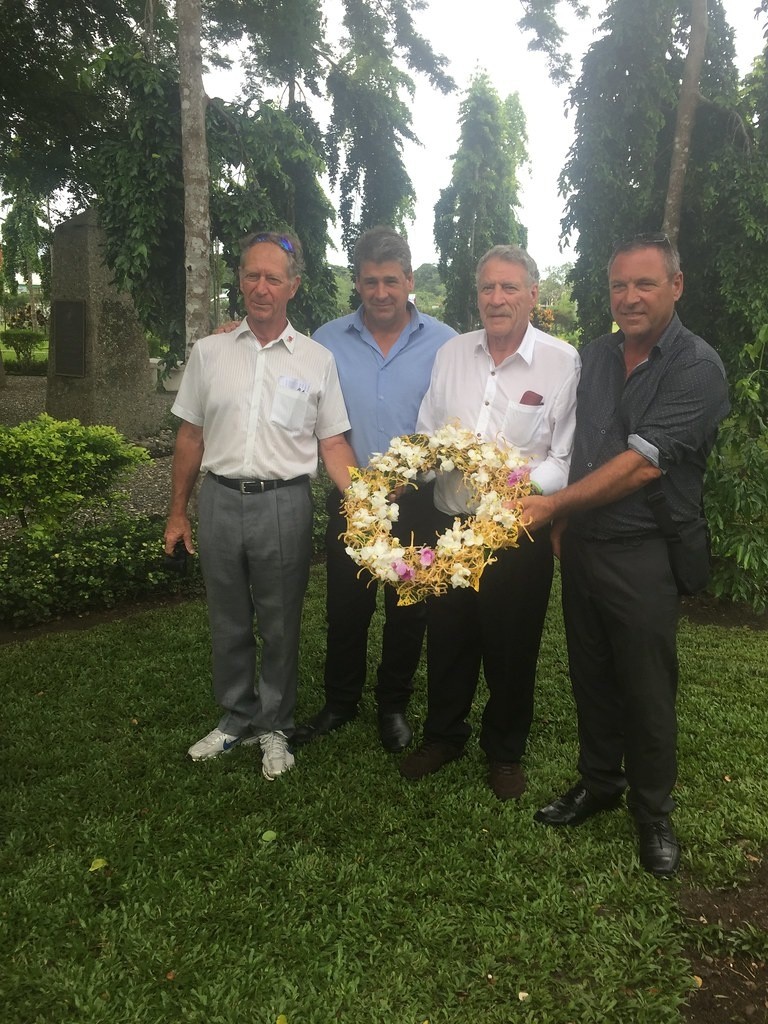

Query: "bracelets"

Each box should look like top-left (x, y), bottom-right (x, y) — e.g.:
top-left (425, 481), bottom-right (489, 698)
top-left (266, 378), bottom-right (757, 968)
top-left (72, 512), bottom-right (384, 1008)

top-left (529, 481), bottom-right (542, 496)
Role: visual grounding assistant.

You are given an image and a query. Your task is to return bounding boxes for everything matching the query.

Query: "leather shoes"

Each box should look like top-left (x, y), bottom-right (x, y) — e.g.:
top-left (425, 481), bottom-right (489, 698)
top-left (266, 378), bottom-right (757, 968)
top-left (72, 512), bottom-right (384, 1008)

top-left (296, 703), bottom-right (359, 734)
top-left (638, 815), bottom-right (682, 880)
top-left (534, 780), bottom-right (622, 826)
top-left (377, 705), bottom-right (413, 753)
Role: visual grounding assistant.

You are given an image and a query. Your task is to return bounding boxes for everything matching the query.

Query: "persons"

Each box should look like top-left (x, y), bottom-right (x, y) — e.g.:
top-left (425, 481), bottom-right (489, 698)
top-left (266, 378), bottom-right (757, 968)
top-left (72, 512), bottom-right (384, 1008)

top-left (401, 245), bottom-right (582, 800)
top-left (501, 231), bottom-right (735, 877)
top-left (163, 233), bottom-right (406, 780)
top-left (212, 225), bottom-right (459, 751)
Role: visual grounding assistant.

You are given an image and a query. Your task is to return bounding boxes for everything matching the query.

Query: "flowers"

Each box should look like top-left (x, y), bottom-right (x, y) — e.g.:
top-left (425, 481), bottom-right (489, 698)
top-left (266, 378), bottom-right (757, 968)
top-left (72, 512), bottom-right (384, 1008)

top-left (337, 422), bottom-right (534, 604)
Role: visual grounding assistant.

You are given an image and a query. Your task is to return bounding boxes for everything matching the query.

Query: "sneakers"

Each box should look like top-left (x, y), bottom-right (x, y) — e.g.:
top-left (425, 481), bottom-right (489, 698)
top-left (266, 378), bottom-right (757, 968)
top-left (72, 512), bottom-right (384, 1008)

top-left (399, 736), bottom-right (465, 780)
top-left (259, 728), bottom-right (295, 782)
top-left (486, 759), bottom-right (525, 801)
top-left (186, 726), bottom-right (242, 762)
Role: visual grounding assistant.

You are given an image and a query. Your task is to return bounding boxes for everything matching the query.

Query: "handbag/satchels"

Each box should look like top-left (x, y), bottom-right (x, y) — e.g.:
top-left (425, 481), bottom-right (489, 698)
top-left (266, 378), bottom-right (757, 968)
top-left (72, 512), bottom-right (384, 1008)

top-left (666, 516), bottom-right (715, 597)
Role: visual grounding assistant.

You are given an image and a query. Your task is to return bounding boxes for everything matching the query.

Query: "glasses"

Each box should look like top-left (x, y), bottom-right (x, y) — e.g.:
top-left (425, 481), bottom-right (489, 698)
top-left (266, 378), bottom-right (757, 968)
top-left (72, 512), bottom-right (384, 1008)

top-left (247, 232), bottom-right (297, 260)
top-left (611, 231), bottom-right (680, 264)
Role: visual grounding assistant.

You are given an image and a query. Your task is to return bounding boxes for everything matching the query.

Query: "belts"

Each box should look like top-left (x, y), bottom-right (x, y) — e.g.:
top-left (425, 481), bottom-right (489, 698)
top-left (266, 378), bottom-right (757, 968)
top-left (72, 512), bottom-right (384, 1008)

top-left (206, 471), bottom-right (310, 494)
top-left (583, 532), bottom-right (667, 539)
